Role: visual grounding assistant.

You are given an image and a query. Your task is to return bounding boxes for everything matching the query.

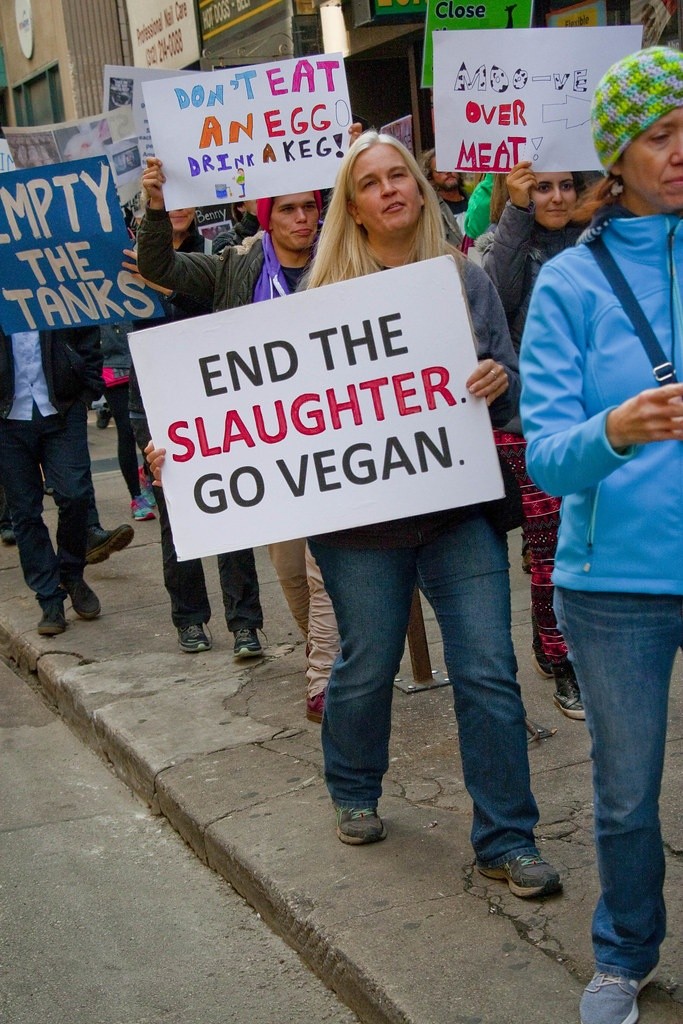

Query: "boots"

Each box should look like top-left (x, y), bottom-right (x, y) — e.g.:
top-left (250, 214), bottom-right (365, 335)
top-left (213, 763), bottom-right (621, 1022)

top-left (550, 666), bottom-right (586, 719)
top-left (531, 634), bottom-right (554, 677)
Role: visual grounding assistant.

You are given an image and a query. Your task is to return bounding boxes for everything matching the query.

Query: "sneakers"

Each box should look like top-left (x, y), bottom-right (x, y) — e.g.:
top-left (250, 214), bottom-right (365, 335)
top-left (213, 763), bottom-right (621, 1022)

top-left (38, 597), bottom-right (66, 634)
top-left (478, 854), bottom-right (563, 897)
top-left (131, 496), bottom-right (156, 520)
top-left (579, 965), bottom-right (659, 1024)
top-left (332, 802), bottom-right (387, 845)
top-left (61, 580), bottom-right (101, 619)
top-left (177, 623), bottom-right (211, 652)
top-left (138, 465), bottom-right (156, 507)
top-left (233, 627), bottom-right (263, 657)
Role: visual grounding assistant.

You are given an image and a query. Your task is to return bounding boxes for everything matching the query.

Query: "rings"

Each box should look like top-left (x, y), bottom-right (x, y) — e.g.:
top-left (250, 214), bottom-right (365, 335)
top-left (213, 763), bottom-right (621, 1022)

top-left (490, 371), bottom-right (498, 379)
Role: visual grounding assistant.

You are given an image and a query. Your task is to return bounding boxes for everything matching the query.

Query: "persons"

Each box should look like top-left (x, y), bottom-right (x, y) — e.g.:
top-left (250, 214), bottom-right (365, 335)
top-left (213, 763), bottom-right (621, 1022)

top-left (144, 132), bottom-right (561, 897)
top-left (519, 47), bottom-right (683, 1024)
top-left (0, 123), bottom-right (591, 724)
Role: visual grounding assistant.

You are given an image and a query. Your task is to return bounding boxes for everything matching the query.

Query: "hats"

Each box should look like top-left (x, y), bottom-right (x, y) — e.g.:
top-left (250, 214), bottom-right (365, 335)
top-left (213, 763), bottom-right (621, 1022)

top-left (590, 46), bottom-right (683, 172)
top-left (256, 190), bottom-right (322, 233)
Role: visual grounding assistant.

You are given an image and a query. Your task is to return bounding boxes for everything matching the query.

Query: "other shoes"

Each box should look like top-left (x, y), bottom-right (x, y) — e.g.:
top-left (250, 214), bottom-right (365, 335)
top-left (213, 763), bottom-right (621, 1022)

top-left (305, 692), bottom-right (325, 722)
top-left (306, 643), bottom-right (310, 657)
top-left (2, 531), bottom-right (15, 544)
top-left (42, 481), bottom-right (53, 495)
top-left (85, 524), bottom-right (135, 564)
top-left (97, 407), bottom-right (111, 429)
top-left (520, 533), bottom-right (532, 572)
